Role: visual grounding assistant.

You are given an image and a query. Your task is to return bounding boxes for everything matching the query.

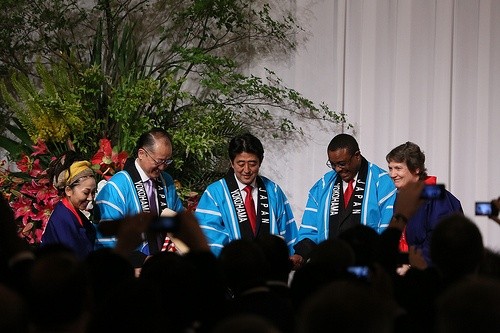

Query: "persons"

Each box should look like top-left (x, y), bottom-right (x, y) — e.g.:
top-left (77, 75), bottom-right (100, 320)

top-left (93, 127), bottom-right (183, 265)
top-left (386, 141), bottom-right (463, 263)
top-left (0, 181), bottom-right (500, 333)
top-left (194, 133), bottom-right (298, 259)
top-left (41, 150), bottom-right (97, 254)
top-left (290, 133), bottom-right (398, 271)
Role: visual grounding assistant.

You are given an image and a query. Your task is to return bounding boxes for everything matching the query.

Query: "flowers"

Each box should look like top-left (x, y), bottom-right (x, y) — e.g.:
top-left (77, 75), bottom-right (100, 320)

top-left (0, 138), bottom-right (198, 253)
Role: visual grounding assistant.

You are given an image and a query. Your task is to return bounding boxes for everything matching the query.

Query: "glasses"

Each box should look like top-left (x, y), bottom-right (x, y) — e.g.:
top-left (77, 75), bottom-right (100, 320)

top-left (326, 154), bottom-right (354, 168)
top-left (139, 147), bottom-right (174, 167)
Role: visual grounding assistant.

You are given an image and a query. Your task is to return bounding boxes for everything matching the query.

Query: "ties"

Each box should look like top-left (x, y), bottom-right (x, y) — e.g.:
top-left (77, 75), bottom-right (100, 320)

top-left (147, 179), bottom-right (161, 251)
top-left (243, 186), bottom-right (257, 236)
top-left (344, 178), bottom-right (355, 209)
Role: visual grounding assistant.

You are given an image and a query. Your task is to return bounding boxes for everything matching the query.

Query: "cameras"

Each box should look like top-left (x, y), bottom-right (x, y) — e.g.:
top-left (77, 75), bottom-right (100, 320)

top-left (149, 216), bottom-right (174, 230)
top-left (475, 202), bottom-right (499, 216)
top-left (349, 266), bottom-right (369, 278)
